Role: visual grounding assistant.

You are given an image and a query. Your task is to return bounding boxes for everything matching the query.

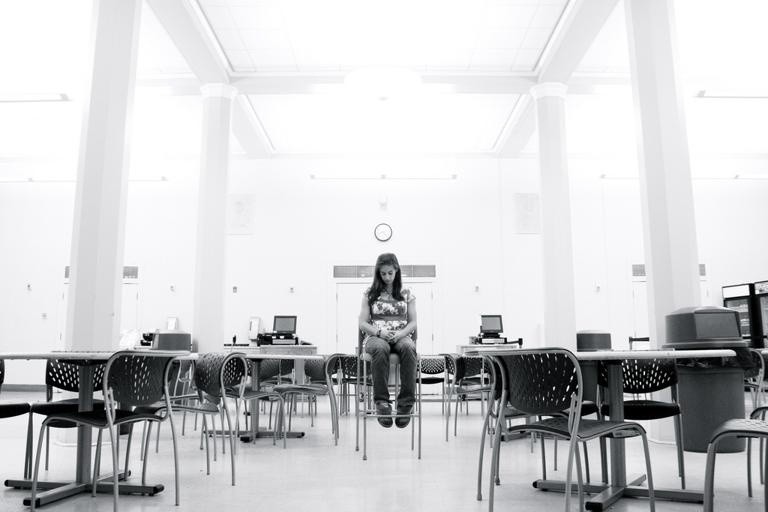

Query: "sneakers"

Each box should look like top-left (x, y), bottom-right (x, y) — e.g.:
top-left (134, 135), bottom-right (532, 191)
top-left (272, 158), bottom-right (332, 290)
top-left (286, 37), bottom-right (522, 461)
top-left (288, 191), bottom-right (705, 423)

top-left (395, 406), bottom-right (411, 428)
top-left (376, 403), bottom-right (392, 427)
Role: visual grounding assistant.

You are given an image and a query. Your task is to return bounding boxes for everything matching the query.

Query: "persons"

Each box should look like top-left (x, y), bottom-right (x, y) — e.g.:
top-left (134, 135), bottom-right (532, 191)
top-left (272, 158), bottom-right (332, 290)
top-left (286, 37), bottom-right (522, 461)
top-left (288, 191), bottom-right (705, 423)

top-left (359, 253), bottom-right (416, 429)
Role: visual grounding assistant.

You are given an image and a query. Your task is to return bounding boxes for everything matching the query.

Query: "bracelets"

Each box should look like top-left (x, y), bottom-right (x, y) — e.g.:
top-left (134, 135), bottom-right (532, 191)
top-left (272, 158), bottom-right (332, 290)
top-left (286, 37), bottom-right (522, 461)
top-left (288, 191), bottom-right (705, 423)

top-left (377, 330), bottom-right (380, 339)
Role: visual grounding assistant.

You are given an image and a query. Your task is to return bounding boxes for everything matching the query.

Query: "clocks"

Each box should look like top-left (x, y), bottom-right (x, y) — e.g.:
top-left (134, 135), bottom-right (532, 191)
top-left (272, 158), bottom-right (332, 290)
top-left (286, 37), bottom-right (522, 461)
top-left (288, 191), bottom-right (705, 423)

top-left (374, 223), bottom-right (392, 242)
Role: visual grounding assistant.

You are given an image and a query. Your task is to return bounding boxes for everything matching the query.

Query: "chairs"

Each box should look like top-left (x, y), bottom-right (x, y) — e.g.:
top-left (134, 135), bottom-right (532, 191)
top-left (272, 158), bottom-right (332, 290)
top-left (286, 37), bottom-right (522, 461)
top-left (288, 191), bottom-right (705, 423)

top-left (355, 328), bottom-right (422, 461)
top-left (489, 347), bottom-right (655, 512)
top-left (747, 405), bottom-right (768, 497)
top-left (443, 352), bottom-right (489, 417)
top-left (744, 350), bottom-right (765, 408)
top-left (446, 355), bottom-right (489, 442)
top-left (598, 348), bottom-right (686, 489)
top-left (416, 353), bottom-right (449, 415)
top-left (703, 419), bottom-right (768, 512)
top-left (477, 352), bottom-right (590, 501)
top-left (0, 344), bottom-right (374, 512)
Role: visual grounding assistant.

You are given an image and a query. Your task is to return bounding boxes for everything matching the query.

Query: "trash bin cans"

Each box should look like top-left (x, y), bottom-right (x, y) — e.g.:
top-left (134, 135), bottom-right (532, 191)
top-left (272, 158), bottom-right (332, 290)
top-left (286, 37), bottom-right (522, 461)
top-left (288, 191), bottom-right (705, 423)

top-left (661, 305), bottom-right (748, 453)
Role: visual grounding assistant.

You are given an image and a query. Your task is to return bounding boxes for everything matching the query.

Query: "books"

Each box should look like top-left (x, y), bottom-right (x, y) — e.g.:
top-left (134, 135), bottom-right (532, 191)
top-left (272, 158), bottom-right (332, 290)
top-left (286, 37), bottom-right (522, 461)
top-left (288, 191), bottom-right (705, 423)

top-left (460, 337), bottom-right (520, 353)
top-left (273, 339), bottom-right (296, 344)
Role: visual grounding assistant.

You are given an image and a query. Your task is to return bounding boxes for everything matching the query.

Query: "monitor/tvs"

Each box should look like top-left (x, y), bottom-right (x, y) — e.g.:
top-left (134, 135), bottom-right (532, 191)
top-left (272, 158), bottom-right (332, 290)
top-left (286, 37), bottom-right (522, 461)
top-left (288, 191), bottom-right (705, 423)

top-left (273, 315), bottom-right (296, 338)
top-left (481, 314), bottom-right (503, 338)
